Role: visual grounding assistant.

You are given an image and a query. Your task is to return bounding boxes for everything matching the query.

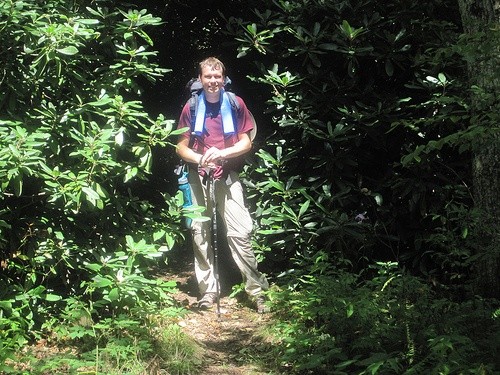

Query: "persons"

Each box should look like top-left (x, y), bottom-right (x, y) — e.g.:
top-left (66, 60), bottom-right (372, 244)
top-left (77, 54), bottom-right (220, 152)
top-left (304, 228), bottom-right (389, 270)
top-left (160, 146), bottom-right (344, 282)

top-left (175, 57), bottom-right (273, 314)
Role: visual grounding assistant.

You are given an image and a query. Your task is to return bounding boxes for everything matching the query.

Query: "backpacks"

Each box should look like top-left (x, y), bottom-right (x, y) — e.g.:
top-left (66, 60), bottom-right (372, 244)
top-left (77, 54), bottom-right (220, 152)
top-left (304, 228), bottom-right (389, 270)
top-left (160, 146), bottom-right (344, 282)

top-left (178, 76), bottom-right (242, 166)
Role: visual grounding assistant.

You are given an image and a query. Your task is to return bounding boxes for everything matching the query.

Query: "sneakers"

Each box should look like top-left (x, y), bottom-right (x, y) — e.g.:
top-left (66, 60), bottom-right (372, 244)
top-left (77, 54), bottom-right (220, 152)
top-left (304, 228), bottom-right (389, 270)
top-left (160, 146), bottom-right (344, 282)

top-left (198, 293), bottom-right (220, 308)
top-left (249, 294), bottom-right (272, 313)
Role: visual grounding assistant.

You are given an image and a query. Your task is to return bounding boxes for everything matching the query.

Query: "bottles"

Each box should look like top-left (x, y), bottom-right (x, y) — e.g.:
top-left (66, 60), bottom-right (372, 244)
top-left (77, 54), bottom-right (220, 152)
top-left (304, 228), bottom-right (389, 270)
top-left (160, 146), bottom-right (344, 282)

top-left (179, 177), bottom-right (192, 207)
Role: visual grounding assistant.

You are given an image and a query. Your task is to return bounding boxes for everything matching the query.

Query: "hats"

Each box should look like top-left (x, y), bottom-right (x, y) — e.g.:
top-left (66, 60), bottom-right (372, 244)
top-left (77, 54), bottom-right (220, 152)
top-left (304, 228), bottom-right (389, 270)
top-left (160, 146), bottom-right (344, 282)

top-left (246, 109), bottom-right (257, 140)
